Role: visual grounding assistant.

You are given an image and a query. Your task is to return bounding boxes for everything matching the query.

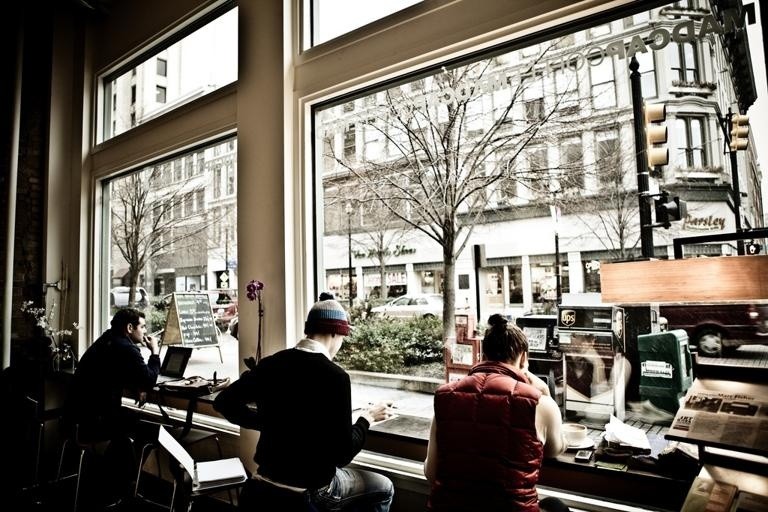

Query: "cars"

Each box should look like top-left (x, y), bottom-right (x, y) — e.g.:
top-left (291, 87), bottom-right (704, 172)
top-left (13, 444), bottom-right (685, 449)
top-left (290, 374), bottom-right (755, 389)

top-left (111, 286), bottom-right (172, 310)
top-left (661, 305), bottom-right (768, 358)
top-left (369, 293), bottom-right (443, 319)
top-left (208, 290), bottom-right (238, 341)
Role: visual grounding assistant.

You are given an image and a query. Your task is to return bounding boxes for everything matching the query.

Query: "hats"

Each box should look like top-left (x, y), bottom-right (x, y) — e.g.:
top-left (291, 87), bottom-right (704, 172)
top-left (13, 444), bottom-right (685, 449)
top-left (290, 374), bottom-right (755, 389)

top-left (304, 292), bottom-right (349, 336)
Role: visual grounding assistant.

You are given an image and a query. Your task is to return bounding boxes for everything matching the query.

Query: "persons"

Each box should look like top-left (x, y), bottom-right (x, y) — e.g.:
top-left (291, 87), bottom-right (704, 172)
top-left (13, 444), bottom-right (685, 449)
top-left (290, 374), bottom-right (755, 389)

top-left (370, 286), bottom-right (379, 297)
top-left (423, 313), bottom-right (566, 512)
top-left (214, 291), bottom-right (395, 512)
top-left (218, 289), bottom-right (231, 300)
top-left (63, 307), bottom-right (161, 509)
top-left (554, 310), bottom-right (632, 422)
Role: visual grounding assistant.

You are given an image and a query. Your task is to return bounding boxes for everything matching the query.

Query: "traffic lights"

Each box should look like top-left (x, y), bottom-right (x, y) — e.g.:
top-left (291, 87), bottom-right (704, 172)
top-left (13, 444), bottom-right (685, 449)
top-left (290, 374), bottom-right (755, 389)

top-left (642, 103), bottom-right (669, 172)
top-left (654, 196), bottom-right (687, 229)
top-left (730, 114), bottom-right (749, 151)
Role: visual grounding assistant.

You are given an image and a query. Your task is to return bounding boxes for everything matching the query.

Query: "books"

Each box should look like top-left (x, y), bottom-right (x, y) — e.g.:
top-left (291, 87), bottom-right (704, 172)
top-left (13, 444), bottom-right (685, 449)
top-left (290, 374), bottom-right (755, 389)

top-left (679, 475), bottom-right (738, 512)
top-left (730, 489), bottom-right (768, 512)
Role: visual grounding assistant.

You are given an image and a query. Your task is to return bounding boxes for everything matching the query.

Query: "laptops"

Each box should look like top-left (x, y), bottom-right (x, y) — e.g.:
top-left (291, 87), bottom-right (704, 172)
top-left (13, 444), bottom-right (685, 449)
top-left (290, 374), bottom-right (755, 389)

top-left (155, 346), bottom-right (193, 385)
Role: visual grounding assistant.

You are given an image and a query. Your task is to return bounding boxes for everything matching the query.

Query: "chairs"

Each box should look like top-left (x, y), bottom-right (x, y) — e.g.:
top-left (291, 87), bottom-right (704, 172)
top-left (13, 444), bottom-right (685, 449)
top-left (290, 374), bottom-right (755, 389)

top-left (24, 395), bottom-right (250, 511)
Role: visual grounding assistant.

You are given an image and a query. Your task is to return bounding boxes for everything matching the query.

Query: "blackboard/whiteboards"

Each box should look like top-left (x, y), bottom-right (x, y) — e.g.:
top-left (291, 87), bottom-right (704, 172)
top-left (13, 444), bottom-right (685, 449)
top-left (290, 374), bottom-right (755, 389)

top-left (174, 292), bottom-right (219, 349)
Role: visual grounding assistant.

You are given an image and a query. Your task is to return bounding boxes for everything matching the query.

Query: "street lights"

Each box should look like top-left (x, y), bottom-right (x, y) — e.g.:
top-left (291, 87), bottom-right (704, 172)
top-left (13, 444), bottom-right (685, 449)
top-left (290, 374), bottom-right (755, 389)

top-left (548, 176), bottom-right (561, 307)
top-left (224, 220), bottom-right (231, 288)
top-left (344, 202), bottom-right (354, 308)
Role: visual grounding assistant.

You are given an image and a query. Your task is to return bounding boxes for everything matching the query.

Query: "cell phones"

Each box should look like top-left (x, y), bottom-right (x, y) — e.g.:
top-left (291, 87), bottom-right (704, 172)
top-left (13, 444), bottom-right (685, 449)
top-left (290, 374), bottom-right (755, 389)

top-left (575, 449), bottom-right (593, 463)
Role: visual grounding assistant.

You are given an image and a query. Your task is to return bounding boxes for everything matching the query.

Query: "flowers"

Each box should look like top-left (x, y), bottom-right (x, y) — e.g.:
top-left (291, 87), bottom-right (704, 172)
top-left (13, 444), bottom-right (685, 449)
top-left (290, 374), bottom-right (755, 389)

top-left (21, 298), bottom-right (84, 373)
top-left (247, 280), bottom-right (265, 364)
